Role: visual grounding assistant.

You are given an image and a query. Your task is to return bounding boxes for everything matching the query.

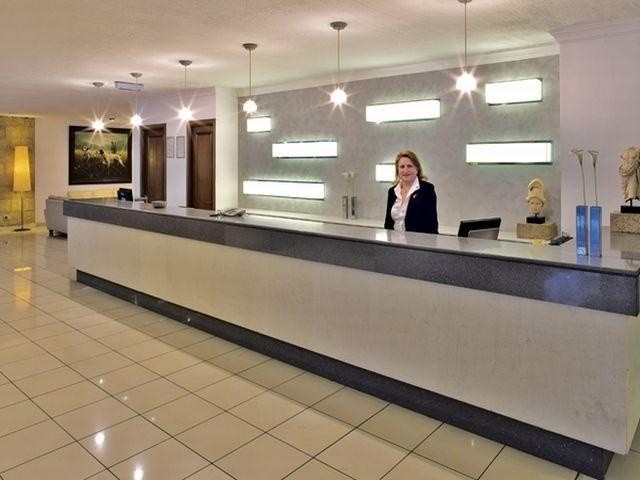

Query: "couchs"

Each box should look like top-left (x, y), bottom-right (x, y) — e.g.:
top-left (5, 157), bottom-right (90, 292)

top-left (45, 187), bottom-right (148, 237)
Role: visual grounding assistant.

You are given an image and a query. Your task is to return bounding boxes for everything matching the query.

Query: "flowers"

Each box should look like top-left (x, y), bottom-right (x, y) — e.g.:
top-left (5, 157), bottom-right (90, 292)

top-left (569, 149), bottom-right (600, 206)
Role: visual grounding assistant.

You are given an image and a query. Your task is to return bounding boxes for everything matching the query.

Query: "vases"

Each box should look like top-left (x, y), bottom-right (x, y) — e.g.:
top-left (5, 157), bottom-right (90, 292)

top-left (577, 205), bottom-right (601, 257)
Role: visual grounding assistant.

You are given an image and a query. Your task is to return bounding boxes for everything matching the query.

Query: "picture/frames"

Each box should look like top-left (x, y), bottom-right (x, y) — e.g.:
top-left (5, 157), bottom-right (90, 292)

top-left (165, 135), bottom-right (186, 158)
top-left (69, 125), bottom-right (132, 185)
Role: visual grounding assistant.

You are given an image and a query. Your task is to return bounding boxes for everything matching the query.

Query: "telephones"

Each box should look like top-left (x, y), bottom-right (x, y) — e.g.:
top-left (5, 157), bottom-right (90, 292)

top-left (221, 207), bottom-right (246, 217)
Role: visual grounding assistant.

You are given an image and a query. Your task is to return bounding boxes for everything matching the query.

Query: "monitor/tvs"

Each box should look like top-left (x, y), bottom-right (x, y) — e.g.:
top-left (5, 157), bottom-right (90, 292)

top-left (457, 215), bottom-right (502, 241)
top-left (117, 187), bottom-right (133, 201)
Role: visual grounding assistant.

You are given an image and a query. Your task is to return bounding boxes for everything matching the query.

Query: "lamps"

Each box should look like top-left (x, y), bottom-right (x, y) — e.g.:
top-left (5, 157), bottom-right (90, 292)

top-left (12, 146), bottom-right (31, 233)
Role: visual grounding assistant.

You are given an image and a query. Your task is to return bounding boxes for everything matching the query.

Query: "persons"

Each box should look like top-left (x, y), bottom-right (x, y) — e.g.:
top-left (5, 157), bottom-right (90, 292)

top-left (524, 179), bottom-right (546, 215)
top-left (619, 145), bottom-right (640, 204)
top-left (386, 149), bottom-right (441, 234)
